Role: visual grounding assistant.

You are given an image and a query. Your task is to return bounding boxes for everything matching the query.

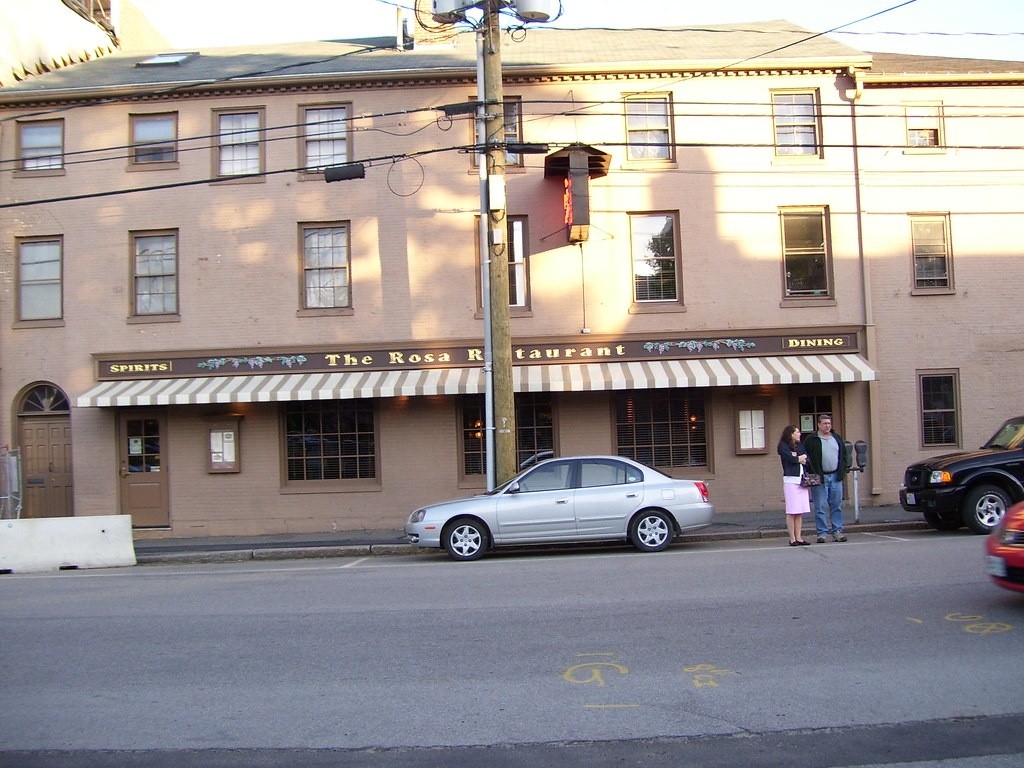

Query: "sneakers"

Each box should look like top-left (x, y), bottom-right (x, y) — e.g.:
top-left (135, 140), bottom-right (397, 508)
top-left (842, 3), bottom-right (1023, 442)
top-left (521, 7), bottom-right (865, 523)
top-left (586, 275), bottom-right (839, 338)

top-left (816, 535), bottom-right (826, 543)
top-left (831, 532), bottom-right (848, 542)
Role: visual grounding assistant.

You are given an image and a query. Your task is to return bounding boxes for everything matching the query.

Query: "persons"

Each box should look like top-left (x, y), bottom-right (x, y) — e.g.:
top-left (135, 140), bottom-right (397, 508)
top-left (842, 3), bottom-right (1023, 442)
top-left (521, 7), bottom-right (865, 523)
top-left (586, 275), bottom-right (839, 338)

top-left (802, 414), bottom-right (849, 543)
top-left (777, 424), bottom-right (811, 546)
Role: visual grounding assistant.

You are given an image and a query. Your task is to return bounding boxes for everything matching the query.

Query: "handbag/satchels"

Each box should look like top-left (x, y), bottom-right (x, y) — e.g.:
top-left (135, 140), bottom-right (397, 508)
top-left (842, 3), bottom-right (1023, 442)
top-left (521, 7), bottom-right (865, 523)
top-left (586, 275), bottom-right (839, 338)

top-left (800, 459), bottom-right (822, 487)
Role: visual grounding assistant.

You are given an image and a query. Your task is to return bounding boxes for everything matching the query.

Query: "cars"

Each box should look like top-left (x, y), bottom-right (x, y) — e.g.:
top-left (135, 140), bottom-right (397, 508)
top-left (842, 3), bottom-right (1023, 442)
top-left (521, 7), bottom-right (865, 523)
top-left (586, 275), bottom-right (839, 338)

top-left (983, 503), bottom-right (1024, 593)
top-left (899, 416), bottom-right (1024, 534)
top-left (520, 451), bottom-right (553, 470)
top-left (406, 455), bottom-right (715, 562)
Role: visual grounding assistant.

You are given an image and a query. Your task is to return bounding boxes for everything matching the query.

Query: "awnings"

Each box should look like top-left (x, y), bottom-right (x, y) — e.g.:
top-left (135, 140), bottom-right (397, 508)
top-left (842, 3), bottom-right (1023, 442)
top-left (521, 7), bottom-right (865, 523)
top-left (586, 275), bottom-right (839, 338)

top-left (75, 351), bottom-right (880, 408)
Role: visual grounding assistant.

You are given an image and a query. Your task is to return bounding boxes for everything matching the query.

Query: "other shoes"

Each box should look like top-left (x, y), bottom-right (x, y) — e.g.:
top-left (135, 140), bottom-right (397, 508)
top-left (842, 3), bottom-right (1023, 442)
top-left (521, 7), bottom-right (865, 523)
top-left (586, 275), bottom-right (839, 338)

top-left (788, 539), bottom-right (799, 546)
top-left (796, 540), bottom-right (811, 545)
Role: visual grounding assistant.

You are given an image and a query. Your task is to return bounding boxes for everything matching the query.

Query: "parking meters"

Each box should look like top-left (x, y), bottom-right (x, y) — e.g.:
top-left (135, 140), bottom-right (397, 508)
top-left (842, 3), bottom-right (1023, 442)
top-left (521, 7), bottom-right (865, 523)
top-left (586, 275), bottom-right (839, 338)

top-left (845, 440), bottom-right (868, 523)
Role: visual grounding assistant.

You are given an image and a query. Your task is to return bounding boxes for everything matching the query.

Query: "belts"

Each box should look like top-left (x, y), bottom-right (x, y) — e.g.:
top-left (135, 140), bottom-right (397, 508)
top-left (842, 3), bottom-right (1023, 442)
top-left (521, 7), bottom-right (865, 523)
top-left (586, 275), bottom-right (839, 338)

top-left (821, 471), bottom-right (837, 474)
